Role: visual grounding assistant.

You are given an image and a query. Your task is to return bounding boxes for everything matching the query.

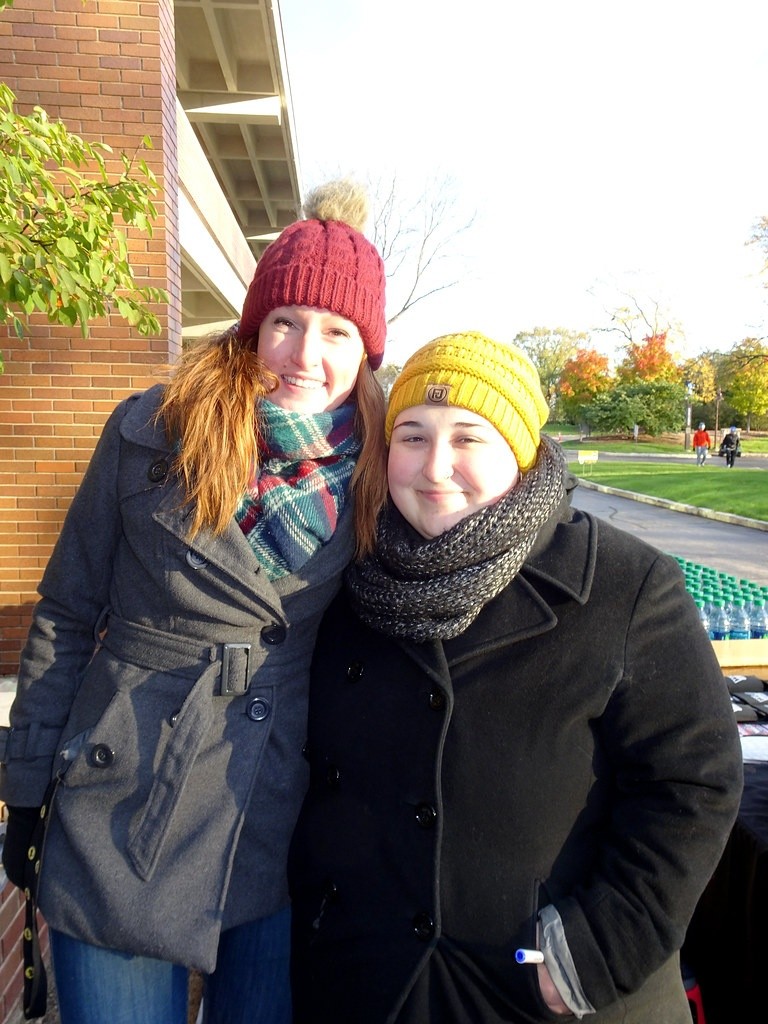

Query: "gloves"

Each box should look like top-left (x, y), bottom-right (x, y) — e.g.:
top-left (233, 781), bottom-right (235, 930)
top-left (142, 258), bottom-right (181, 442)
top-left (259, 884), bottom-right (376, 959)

top-left (2, 803), bottom-right (44, 893)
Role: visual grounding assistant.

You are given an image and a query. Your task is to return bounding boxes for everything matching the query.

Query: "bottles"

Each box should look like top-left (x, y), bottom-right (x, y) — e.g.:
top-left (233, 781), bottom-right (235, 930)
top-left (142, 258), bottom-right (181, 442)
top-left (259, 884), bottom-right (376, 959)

top-left (667, 553), bottom-right (768, 641)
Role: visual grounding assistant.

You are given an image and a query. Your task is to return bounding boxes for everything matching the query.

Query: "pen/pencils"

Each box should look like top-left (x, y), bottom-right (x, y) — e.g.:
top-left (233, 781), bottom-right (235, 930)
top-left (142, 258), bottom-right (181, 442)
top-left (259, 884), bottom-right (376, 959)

top-left (514, 948), bottom-right (545, 965)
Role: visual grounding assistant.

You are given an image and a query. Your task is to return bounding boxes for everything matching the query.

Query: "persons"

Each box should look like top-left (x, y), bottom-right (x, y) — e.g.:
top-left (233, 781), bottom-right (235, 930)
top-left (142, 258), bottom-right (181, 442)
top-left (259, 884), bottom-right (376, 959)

top-left (692, 422), bottom-right (712, 468)
top-left (287, 328), bottom-right (744, 1024)
top-left (720, 426), bottom-right (740, 469)
top-left (1, 177), bottom-right (385, 1024)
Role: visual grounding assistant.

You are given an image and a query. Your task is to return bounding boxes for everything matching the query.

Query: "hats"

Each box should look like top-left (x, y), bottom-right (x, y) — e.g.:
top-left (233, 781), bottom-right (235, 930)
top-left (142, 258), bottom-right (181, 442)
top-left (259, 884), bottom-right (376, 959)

top-left (385, 332), bottom-right (550, 477)
top-left (699, 423), bottom-right (705, 429)
top-left (238, 179), bottom-right (387, 372)
top-left (731, 426), bottom-right (736, 432)
